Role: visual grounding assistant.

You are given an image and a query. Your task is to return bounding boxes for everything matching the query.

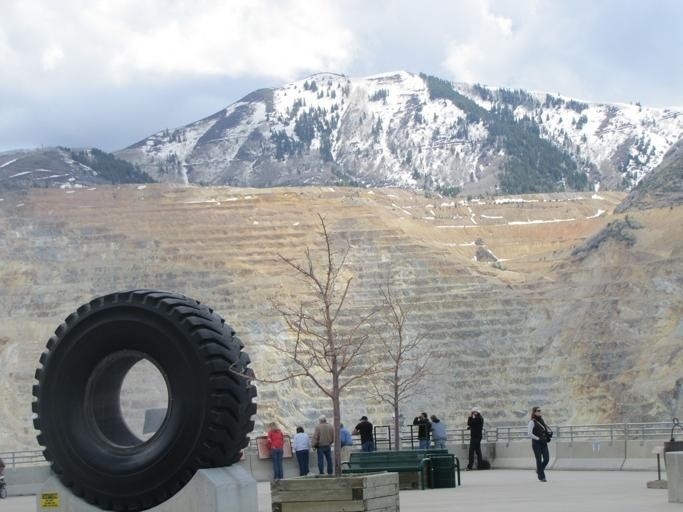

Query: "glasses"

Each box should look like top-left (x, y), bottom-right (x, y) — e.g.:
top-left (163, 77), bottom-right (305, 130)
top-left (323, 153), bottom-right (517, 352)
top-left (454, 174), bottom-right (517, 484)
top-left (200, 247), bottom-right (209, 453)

top-left (537, 410), bottom-right (541, 412)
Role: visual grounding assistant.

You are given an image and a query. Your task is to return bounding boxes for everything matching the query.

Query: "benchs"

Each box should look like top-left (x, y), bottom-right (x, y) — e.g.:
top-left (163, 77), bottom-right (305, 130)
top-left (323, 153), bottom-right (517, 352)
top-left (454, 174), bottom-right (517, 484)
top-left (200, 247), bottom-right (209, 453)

top-left (340, 450), bottom-right (460, 490)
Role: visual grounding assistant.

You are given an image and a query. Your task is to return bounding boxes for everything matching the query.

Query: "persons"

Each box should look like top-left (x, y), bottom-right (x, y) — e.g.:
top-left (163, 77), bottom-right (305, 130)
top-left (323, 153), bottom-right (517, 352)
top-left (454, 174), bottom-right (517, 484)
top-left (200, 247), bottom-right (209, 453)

top-left (267, 422), bottom-right (284, 479)
top-left (312, 415), bottom-right (335, 475)
top-left (292, 427), bottom-right (310, 476)
top-left (464, 407), bottom-right (483, 470)
top-left (352, 416), bottom-right (374, 451)
top-left (0, 458), bottom-right (5, 477)
top-left (340, 423), bottom-right (352, 469)
top-left (430, 414), bottom-right (448, 449)
top-left (528, 406), bottom-right (549, 481)
top-left (414, 413), bottom-right (432, 448)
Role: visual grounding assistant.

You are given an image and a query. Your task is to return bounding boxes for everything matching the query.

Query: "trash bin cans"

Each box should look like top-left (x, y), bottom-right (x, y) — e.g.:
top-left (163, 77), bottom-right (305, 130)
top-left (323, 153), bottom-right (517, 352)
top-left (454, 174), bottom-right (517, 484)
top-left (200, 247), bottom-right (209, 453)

top-left (426, 454), bottom-right (455, 489)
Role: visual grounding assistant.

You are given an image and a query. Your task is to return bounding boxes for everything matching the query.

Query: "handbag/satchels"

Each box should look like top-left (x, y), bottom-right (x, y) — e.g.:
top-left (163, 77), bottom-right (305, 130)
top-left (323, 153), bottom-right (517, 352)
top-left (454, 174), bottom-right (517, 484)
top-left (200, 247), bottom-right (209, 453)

top-left (541, 430), bottom-right (551, 442)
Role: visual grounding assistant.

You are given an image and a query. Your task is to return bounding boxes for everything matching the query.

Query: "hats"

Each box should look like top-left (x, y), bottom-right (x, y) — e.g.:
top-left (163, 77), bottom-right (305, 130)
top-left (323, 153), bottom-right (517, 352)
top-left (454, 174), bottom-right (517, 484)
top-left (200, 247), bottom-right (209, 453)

top-left (360, 416), bottom-right (367, 421)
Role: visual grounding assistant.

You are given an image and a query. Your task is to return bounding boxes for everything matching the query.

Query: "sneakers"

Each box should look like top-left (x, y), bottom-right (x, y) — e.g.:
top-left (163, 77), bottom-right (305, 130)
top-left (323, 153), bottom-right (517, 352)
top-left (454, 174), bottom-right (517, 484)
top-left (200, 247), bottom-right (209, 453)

top-left (536, 469), bottom-right (546, 482)
